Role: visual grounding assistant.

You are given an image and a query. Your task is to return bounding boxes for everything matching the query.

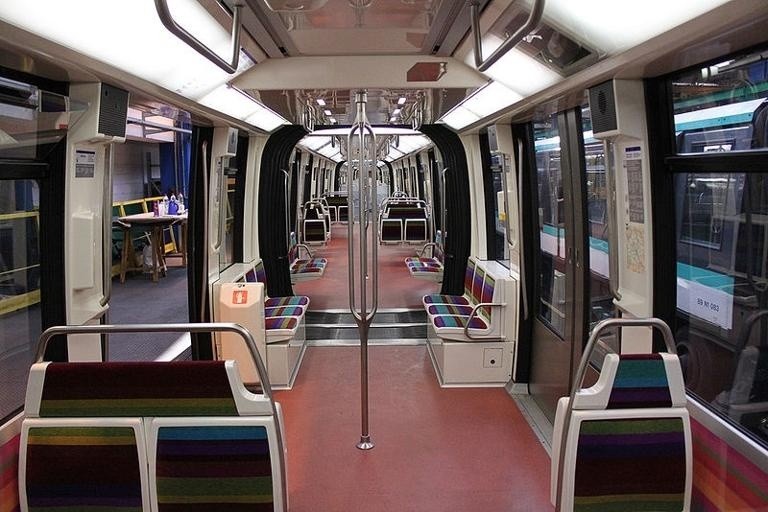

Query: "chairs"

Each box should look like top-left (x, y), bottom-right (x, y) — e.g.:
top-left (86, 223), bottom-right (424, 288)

top-left (402, 229), bottom-right (448, 293)
top-left (550, 317), bottom-right (692, 512)
top-left (421, 255), bottom-right (521, 388)
top-left (212, 258), bottom-right (311, 389)
top-left (303, 190), bottom-right (349, 246)
top-left (15, 322), bottom-right (288, 512)
top-left (377, 190), bottom-right (432, 246)
top-left (288, 231), bottom-right (328, 295)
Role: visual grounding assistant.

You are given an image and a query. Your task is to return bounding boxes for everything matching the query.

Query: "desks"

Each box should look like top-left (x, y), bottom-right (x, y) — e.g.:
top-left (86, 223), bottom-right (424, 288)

top-left (118, 209), bottom-right (188, 284)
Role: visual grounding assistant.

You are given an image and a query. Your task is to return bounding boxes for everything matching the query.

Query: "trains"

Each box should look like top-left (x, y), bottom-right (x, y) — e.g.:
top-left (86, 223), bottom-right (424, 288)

top-left (491, 82), bottom-right (768, 443)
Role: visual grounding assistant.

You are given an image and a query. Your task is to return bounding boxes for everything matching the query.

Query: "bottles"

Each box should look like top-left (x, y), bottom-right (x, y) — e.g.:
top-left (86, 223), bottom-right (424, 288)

top-left (168, 200), bottom-right (179, 215)
top-left (163, 192), bottom-right (184, 206)
